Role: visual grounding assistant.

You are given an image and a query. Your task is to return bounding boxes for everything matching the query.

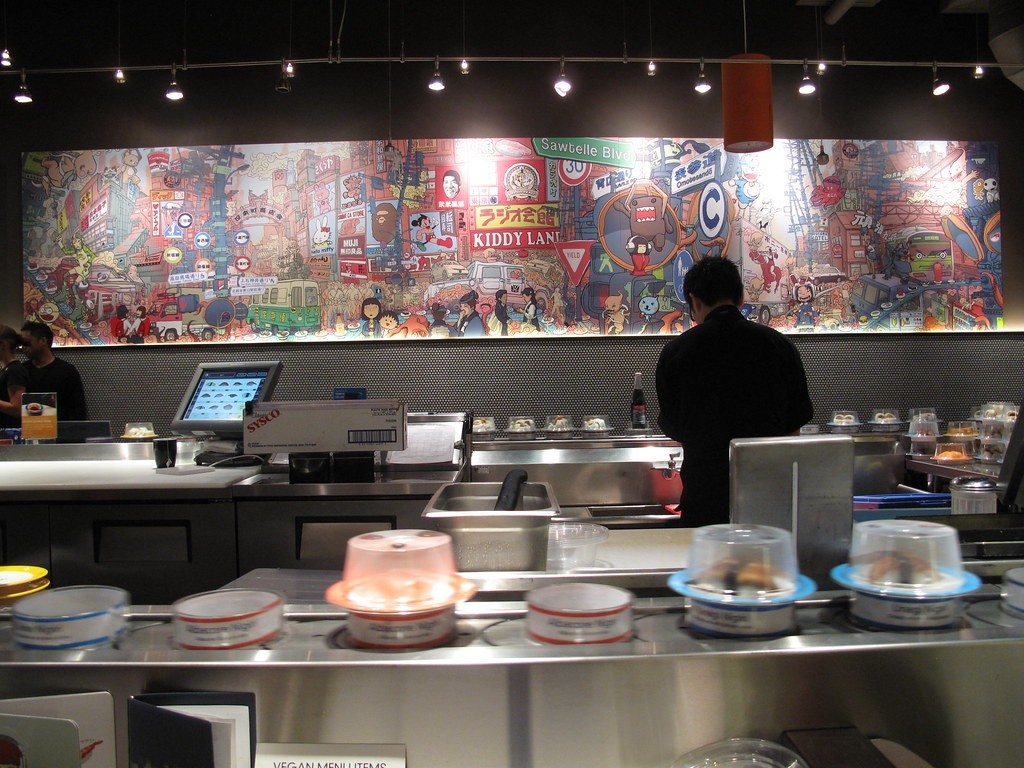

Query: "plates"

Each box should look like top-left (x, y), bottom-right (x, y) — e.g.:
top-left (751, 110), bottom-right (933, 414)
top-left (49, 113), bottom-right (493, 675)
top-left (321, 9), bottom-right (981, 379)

top-left (666, 568), bottom-right (817, 605)
top-left (473, 427), bottom-right (615, 432)
top-left (826, 419), bottom-right (1016, 461)
top-left (0, 565), bottom-right (51, 616)
top-left (828, 563), bottom-right (981, 599)
top-left (325, 573), bottom-right (477, 614)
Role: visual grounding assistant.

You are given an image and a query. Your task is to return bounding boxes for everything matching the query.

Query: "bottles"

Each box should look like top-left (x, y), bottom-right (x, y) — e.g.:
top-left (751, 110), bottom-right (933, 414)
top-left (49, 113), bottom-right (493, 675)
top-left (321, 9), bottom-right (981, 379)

top-left (630, 373), bottom-right (647, 429)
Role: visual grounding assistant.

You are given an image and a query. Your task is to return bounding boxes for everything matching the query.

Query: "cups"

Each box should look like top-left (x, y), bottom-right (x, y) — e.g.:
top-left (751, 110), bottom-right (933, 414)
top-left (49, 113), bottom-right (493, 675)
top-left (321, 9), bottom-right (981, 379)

top-left (153, 437), bottom-right (177, 469)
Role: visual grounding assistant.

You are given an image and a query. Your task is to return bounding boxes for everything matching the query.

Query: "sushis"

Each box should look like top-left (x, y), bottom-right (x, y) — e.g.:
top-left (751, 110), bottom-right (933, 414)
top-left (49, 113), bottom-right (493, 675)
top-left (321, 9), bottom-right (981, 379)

top-left (870, 555), bottom-right (938, 583)
top-left (973, 409), bottom-right (1018, 461)
top-left (833, 412), bottom-right (897, 424)
top-left (699, 558), bottom-right (778, 591)
top-left (473, 417), bottom-right (606, 432)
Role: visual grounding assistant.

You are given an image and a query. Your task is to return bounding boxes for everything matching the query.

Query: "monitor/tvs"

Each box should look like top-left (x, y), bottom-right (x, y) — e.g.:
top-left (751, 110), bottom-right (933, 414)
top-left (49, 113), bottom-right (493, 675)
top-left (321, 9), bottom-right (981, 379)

top-left (170, 360), bottom-right (284, 435)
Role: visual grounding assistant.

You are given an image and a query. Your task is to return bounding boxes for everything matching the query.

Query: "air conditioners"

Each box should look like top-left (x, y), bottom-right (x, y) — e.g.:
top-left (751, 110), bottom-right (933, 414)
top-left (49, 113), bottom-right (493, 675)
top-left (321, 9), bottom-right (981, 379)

top-left (427, 70), bottom-right (443, 91)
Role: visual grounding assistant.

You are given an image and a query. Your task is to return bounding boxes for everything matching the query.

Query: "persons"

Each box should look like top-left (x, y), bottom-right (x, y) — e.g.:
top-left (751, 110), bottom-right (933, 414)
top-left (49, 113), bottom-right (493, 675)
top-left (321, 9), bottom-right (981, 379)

top-left (0, 321), bottom-right (86, 443)
top-left (655, 256), bottom-right (814, 529)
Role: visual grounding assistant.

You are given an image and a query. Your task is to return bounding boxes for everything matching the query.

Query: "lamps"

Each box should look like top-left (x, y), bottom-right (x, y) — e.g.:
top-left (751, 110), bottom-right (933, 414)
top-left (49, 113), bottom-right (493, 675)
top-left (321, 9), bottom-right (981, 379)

top-left (115, 10), bottom-right (126, 83)
top-left (720, 0), bottom-right (775, 153)
top-left (275, 71), bottom-right (291, 94)
top-left (931, 68), bottom-right (951, 97)
top-left (554, 67), bottom-right (571, 95)
top-left (13, 78), bottom-right (33, 103)
top-left (973, 0), bottom-right (984, 79)
top-left (384, 0), bottom-right (396, 153)
top-left (798, 67), bottom-right (816, 95)
top-left (694, 69), bottom-right (711, 92)
top-left (164, 75), bottom-right (184, 98)
top-left (0, 0), bottom-right (12, 66)
top-left (459, 0), bottom-right (471, 75)
top-left (817, 0), bottom-right (828, 166)
